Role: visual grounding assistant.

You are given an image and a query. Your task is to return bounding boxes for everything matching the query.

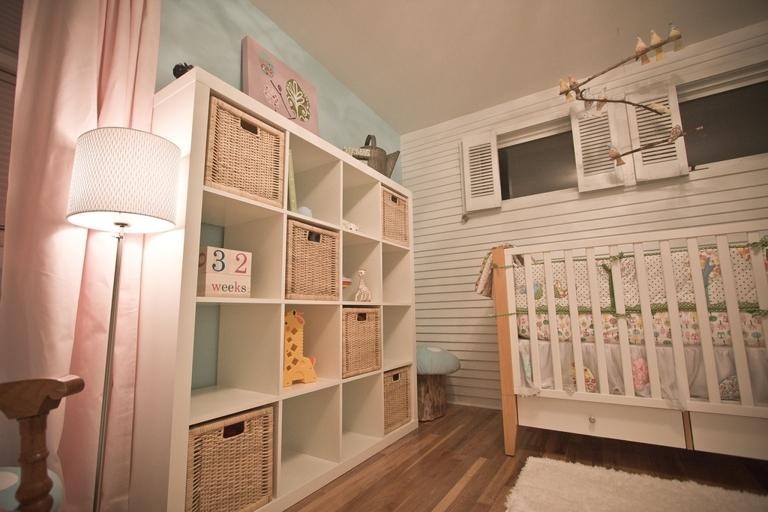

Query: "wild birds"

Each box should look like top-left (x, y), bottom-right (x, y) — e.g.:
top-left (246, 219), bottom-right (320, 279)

top-left (584, 87), bottom-right (594, 111)
top-left (606, 144), bottom-right (625, 167)
top-left (649, 29), bottom-right (663, 61)
top-left (650, 105), bottom-right (668, 115)
top-left (668, 124), bottom-right (681, 144)
top-left (597, 86), bottom-right (608, 111)
top-left (668, 21), bottom-right (682, 52)
top-left (568, 76), bottom-right (582, 100)
top-left (559, 79), bottom-right (572, 101)
top-left (635, 36), bottom-right (650, 66)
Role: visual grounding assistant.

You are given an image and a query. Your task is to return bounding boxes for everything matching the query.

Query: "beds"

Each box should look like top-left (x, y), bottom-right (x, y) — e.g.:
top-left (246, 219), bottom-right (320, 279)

top-left (475, 218), bottom-right (767, 471)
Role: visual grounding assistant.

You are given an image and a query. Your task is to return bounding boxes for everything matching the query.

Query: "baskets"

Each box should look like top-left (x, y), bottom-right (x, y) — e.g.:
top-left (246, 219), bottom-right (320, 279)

top-left (204, 96), bottom-right (285, 209)
top-left (342, 308), bottom-right (382, 379)
top-left (360, 134), bottom-right (388, 177)
top-left (381, 184), bottom-right (409, 248)
top-left (286, 219), bottom-right (340, 301)
top-left (384, 365), bottom-right (411, 434)
top-left (185, 406), bottom-right (273, 512)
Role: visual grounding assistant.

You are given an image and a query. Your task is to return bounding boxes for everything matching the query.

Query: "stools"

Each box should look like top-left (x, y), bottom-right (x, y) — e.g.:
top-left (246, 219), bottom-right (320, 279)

top-left (417, 339), bottom-right (460, 422)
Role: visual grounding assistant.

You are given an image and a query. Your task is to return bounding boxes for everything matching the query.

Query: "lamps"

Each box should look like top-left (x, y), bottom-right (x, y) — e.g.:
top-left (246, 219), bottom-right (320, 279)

top-left (64, 121), bottom-right (186, 510)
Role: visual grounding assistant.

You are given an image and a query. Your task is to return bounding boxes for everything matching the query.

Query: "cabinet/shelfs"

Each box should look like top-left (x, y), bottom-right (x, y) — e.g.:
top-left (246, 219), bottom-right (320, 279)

top-left (123, 67), bottom-right (419, 512)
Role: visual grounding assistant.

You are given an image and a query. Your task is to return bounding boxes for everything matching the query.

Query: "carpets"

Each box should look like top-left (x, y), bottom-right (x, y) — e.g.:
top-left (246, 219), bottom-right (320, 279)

top-left (500, 450), bottom-right (767, 512)
